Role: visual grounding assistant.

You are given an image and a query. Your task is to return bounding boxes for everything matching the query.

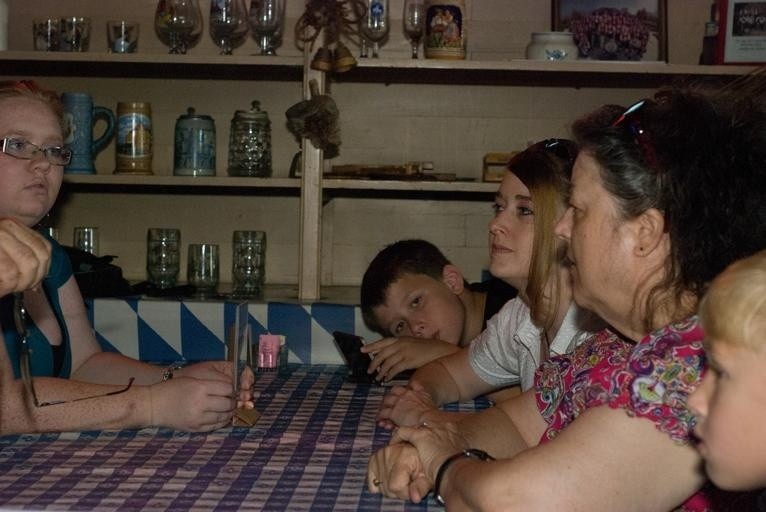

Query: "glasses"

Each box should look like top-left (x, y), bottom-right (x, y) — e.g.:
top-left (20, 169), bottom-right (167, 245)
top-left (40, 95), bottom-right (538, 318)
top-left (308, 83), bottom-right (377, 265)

top-left (12, 252), bottom-right (137, 408)
top-left (542, 133), bottom-right (573, 182)
top-left (614, 94), bottom-right (695, 172)
top-left (0, 135), bottom-right (72, 167)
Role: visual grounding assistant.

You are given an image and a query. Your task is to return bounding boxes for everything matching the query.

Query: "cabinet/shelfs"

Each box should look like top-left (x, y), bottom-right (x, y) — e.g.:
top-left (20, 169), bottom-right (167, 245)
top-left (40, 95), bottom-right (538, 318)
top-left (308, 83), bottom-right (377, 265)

top-left (0, 50), bottom-right (766, 366)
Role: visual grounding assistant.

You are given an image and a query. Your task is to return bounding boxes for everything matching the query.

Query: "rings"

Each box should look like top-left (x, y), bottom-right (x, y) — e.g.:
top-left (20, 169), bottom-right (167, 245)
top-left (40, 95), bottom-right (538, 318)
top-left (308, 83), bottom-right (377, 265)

top-left (372, 478), bottom-right (382, 486)
top-left (418, 422), bottom-right (429, 429)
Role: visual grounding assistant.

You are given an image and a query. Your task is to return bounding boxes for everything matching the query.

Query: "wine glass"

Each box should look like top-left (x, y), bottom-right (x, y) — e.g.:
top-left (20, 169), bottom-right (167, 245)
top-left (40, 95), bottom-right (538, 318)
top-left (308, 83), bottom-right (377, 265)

top-left (360, 1), bottom-right (388, 58)
top-left (249, 0), bottom-right (286, 56)
top-left (402, 1), bottom-right (425, 59)
top-left (154, 0), bottom-right (204, 54)
top-left (358, 36), bottom-right (369, 57)
top-left (208, 0), bottom-right (251, 56)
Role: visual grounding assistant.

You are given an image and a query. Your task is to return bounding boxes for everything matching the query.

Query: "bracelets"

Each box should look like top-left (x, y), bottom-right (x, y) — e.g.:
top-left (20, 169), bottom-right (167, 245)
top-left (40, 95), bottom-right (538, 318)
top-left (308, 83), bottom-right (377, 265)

top-left (434, 449), bottom-right (498, 507)
top-left (161, 364), bottom-right (182, 383)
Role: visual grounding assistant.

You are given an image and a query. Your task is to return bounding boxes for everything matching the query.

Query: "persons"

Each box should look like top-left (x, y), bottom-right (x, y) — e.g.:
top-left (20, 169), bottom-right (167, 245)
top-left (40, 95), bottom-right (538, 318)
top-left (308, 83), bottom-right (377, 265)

top-left (0, 218), bottom-right (53, 299)
top-left (685, 248), bottom-right (766, 494)
top-left (375, 137), bottom-right (598, 434)
top-left (367, 92), bottom-right (766, 511)
top-left (359, 239), bottom-right (519, 383)
top-left (0, 87), bottom-right (257, 438)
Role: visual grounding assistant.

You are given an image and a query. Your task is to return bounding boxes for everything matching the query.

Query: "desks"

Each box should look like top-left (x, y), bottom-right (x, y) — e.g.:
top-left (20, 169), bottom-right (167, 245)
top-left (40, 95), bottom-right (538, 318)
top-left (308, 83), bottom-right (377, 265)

top-left (1, 362), bottom-right (495, 512)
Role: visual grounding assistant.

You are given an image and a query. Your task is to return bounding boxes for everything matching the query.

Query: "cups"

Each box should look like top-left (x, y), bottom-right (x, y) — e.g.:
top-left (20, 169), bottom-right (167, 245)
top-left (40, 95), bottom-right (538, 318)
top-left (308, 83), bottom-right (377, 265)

top-left (106, 19), bottom-right (140, 53)
top-left (32, 15), bottom-right (61, 51)
top-left (232, 226), bottom-right (266, 297)
top-left (58, 91), bottom-right (115, 173)
top-left (60, 15), bottom-right (92, 52)
top-left (145, 227), bottom-right (181, 288)
top-left (72, 224), bottom-right (99, 255)
top-left (112, 101), bottom-right (154, 176)
top-left (185, 242), bottom-right (221, 300)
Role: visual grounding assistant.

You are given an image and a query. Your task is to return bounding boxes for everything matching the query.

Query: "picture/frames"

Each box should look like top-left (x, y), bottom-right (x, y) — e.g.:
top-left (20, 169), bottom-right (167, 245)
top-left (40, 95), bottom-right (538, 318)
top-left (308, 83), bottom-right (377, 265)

top-left (718, 0), bottom-right (766, 65)
top-left (551, 0), bottom-right (668, 64)
